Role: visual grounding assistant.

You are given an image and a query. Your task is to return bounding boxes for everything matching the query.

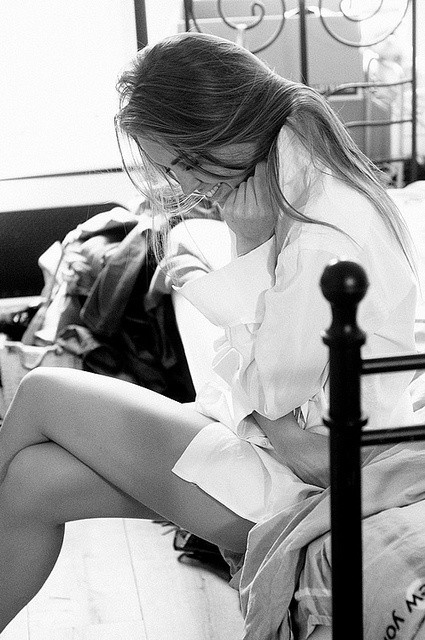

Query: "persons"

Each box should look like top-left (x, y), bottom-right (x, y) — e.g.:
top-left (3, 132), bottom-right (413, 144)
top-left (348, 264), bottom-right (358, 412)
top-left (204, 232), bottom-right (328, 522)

top-left (0, 32), bottom-right (420, 636)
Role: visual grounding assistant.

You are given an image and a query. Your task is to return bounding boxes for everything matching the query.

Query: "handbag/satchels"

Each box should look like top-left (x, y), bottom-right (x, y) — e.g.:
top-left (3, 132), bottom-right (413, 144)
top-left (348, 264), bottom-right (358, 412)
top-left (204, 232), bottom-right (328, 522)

top-left (0, 334), bottom-right (84, 422)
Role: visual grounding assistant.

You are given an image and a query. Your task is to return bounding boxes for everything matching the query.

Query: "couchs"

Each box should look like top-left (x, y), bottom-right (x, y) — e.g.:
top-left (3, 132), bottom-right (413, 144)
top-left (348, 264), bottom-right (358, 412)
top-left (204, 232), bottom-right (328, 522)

top-left (0, 203), bottom-right (144, 340)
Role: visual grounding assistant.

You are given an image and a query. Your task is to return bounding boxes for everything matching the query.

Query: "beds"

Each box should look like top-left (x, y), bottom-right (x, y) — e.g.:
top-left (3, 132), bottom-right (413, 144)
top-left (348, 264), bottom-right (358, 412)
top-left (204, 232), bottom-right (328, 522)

top-left (130, 0), bottom-right (425, 640)
